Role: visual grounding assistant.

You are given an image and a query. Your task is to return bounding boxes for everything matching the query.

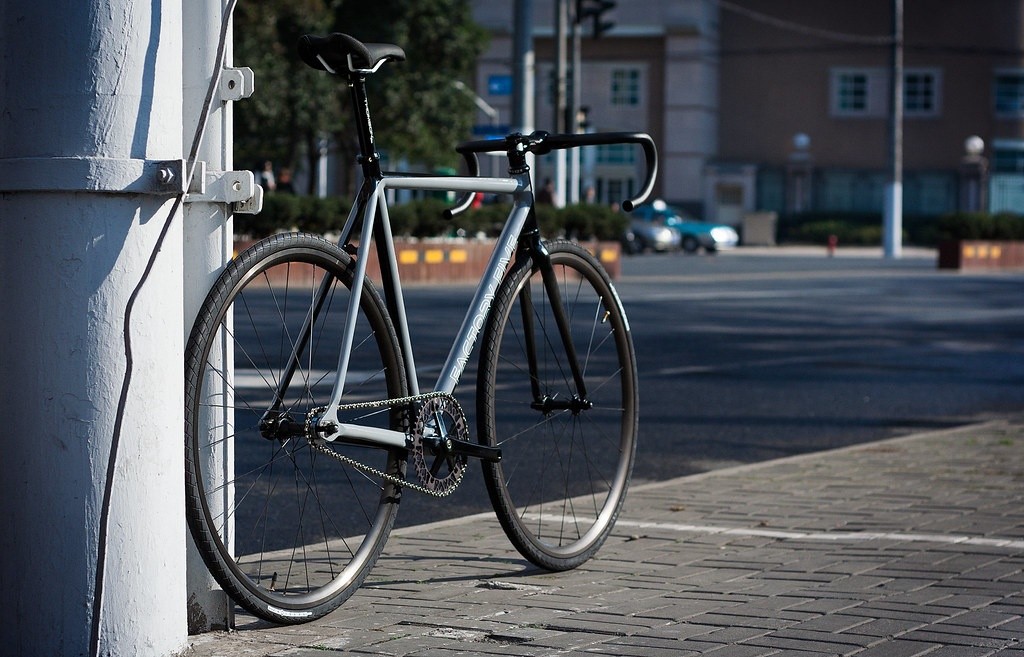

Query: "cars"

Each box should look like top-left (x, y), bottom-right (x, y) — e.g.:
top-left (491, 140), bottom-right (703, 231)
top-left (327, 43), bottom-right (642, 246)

top-left (628, 215), bottom-right (681, 253)
top-left (625, 197), bottom-right (739, 252)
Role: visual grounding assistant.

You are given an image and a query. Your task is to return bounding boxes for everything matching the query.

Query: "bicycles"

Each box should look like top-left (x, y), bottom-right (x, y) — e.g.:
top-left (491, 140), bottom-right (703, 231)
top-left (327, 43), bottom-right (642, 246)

top-left (183, 32), bottom-right (661, 625)
